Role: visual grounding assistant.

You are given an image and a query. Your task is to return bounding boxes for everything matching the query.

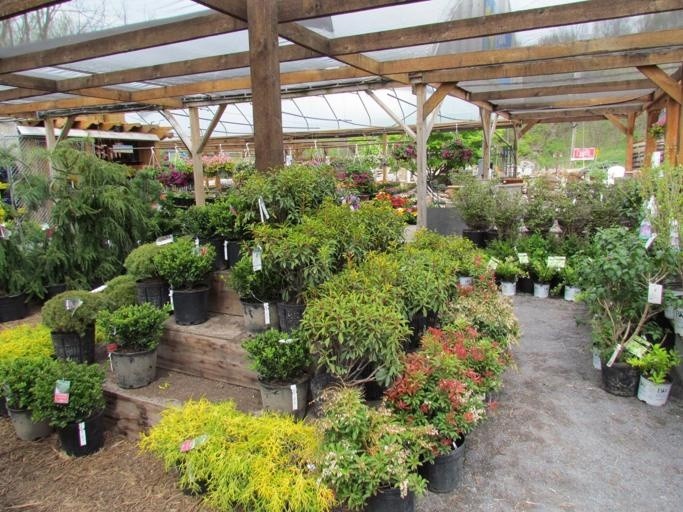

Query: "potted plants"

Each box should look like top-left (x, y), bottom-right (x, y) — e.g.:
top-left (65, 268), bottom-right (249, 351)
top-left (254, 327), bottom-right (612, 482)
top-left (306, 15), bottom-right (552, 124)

top-left (132, 192), bottom-right (518, 510)
top-left (38, 288), bottom-right (101, 369)
top-left (152, 153), bottom-right (588, 301)
top-left (24, 353), bottom-right (110, 456)
top-left (577, 169), bottom-right (681, 410)
top-left (0, 350), bottom-right (54, 441)
top-left (93, 301), bottom-right (173, 388)
top-left (0, 136), bottom-right (244, 322)
